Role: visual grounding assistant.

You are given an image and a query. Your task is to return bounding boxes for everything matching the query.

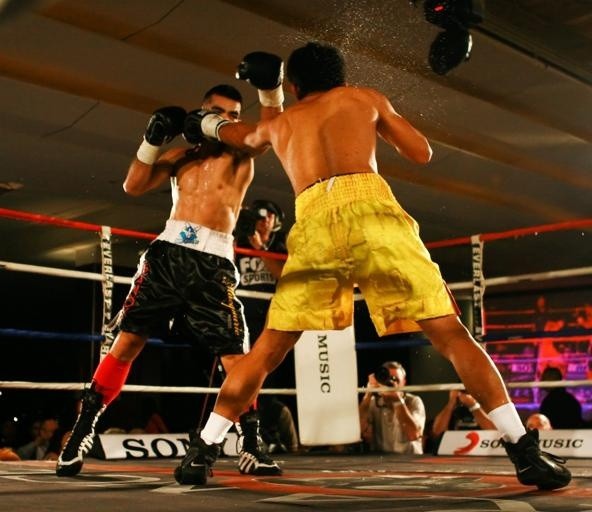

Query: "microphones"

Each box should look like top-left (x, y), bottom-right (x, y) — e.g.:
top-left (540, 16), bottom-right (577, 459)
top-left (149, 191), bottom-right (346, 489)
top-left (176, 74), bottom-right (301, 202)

top-left (268, 224), bottom-right (284, 234)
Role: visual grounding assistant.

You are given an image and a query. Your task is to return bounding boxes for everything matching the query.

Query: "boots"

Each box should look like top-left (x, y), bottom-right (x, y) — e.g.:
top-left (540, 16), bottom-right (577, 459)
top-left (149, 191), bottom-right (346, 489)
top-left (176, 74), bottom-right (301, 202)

top-left (234, 413), bottom-right (284, 476)
top-left (56, 381), bottom-right (107, 474)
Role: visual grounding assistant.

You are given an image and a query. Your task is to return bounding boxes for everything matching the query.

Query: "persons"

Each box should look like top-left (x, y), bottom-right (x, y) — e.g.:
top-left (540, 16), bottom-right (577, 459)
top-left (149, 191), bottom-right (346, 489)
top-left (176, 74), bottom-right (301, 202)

top-left (0, 404), bottom-right (164, 461)
top-left (173, 42), bottom-right (571, 491)
top-left (524, 412), bottom-right (552, 430)
top-left (539, 367), bottom-right (582, 428)
top-left (54, 51), bottom-right (287, 476)
top-left (430, 389), bottom-right (498, 439)
top-left (230, 200), bottom-right (300, 454)
top-left (355, 360), bottom-right (426, 456)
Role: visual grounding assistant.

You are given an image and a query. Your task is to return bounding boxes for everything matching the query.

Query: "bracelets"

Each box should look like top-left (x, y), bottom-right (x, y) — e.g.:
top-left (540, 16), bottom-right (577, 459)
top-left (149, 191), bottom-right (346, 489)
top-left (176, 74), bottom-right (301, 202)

top-left (469, 402), bottom-right (480, 412)
top-left (392, 399), bottom-right (405, 407)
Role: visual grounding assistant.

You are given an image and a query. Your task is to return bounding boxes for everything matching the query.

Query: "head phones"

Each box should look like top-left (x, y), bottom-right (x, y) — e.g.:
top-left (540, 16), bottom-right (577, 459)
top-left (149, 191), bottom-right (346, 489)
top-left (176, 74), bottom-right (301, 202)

top-left (251, 198), bottom-right (286, 226)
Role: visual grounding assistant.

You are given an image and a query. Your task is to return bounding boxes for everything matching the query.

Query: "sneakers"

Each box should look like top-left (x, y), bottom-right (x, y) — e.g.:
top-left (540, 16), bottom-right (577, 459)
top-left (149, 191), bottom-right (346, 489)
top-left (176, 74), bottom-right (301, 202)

top-left (174, 432), bottom-right (227, 485)
top-left (500, 429), bottom-right (572, 490)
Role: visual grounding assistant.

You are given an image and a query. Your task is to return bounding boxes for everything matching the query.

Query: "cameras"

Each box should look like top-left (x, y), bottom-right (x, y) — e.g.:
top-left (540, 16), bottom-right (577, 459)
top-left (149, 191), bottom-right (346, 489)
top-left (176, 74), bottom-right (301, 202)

top-left (366, 359), bottom-right (392, 388)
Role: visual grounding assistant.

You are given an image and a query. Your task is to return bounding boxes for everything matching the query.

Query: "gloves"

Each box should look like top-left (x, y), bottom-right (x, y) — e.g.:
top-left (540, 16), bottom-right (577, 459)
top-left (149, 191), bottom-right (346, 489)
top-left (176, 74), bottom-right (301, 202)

top-left (136, 106), bottom-right (184, 165)
top-left (184, 108), bottom-right (229, 147)
top-left (236, 51), bottom-right (287, 108)
